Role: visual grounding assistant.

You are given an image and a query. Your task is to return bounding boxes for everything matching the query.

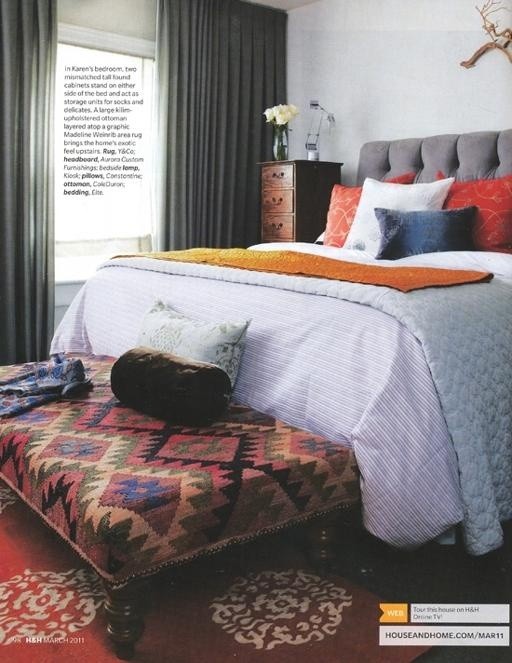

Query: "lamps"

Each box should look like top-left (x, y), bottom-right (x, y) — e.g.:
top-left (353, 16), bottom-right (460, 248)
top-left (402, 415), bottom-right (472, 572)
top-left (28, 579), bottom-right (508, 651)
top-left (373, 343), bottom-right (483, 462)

top-left (305, 101), bottom-right (337, 161)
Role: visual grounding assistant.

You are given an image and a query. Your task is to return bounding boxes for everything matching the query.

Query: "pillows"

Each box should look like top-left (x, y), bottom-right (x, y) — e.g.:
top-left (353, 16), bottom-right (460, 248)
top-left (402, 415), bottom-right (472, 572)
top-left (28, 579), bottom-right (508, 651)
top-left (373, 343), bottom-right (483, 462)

top-left (107, 299), bottom-right (252, 425)
top-left (315, 170), bottom-right (512, 261)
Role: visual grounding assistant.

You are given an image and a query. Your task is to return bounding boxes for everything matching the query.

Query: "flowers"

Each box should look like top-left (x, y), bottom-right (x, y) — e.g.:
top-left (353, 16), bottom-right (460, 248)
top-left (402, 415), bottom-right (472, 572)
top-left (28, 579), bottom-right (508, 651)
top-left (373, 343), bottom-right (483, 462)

top-left (263, 104), bottom-right (298, 159)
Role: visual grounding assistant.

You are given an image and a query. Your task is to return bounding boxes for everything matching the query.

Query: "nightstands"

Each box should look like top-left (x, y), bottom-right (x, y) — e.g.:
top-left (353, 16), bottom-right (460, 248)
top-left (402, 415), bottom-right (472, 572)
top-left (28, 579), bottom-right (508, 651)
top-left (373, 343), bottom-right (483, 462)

top-left (256, 159), bottom-right (344, 243)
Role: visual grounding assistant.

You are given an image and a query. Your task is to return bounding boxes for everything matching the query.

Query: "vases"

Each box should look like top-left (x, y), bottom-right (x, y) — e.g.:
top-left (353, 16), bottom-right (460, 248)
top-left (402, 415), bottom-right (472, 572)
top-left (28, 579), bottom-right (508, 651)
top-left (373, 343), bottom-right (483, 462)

top-left (272, 130), bottom-right (289, 159)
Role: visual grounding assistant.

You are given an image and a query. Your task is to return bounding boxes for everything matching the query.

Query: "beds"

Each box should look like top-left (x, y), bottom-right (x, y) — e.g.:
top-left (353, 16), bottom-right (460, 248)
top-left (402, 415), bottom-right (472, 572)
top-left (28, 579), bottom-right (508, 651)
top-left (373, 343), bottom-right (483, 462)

top-left (0, 352), bottom-right (363, 660)
top-left (69, 133), bottom-right (511, 548)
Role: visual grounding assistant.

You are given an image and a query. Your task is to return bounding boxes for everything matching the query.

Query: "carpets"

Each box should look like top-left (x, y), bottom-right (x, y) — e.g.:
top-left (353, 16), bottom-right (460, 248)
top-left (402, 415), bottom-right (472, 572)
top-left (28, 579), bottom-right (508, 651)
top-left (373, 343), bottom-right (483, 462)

top-left (0, 483), bottom-right (432, 663)
